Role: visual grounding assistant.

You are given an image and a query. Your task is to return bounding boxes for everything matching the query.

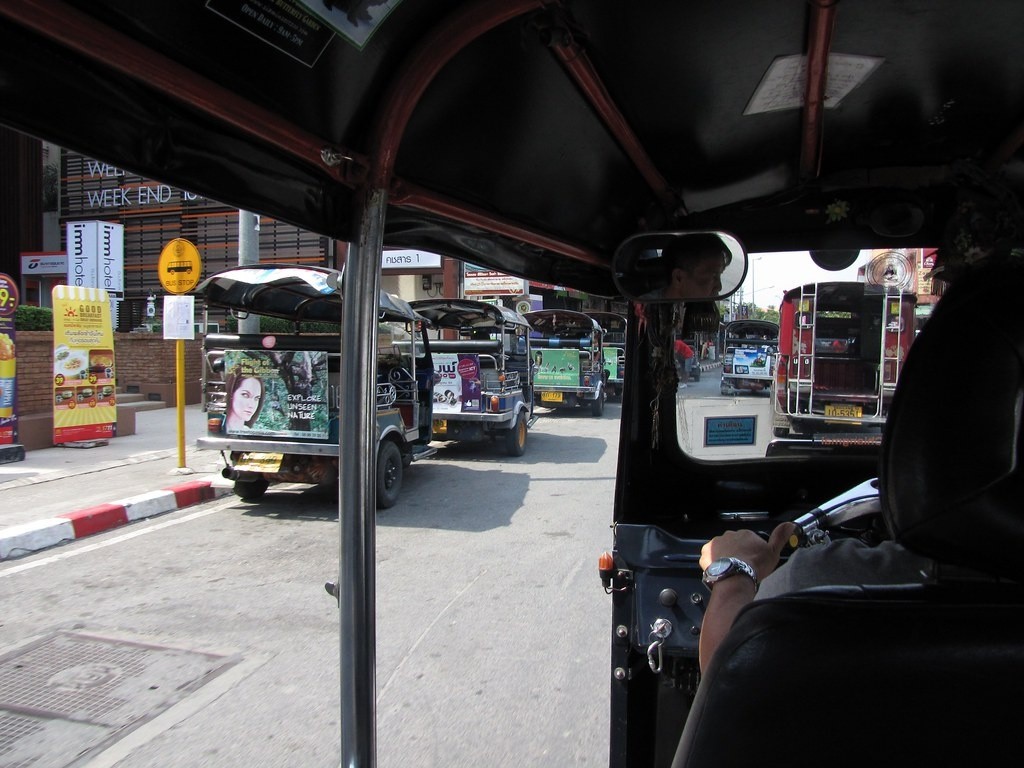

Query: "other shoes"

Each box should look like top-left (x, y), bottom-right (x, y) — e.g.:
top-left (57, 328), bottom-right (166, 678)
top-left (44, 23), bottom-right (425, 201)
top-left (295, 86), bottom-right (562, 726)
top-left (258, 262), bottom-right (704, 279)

top-left (680, 383), bottom-right (687, 389)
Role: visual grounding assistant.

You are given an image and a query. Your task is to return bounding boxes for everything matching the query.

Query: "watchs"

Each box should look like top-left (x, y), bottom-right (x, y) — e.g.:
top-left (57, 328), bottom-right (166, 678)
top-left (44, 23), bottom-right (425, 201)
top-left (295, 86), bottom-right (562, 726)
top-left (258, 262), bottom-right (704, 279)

top-left (701, 557), bottom-right (758, 592)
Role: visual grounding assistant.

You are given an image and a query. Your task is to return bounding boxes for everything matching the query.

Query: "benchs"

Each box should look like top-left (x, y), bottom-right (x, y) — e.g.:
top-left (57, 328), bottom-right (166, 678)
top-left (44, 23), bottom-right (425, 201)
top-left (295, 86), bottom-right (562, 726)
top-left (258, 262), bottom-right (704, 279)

top-left (861, 362), bottom-right (891, 393)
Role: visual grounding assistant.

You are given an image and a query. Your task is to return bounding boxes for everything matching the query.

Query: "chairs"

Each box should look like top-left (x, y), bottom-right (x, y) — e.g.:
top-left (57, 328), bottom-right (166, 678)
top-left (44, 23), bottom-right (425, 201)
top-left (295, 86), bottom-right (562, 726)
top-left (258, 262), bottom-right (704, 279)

top-left (668, 265), bottom-right (1024, 768)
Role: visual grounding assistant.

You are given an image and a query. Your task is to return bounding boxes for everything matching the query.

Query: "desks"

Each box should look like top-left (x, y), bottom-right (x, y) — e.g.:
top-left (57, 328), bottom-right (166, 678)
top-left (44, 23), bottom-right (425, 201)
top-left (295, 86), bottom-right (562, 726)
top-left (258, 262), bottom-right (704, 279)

top-left (815, 357), bottom-right (869, 388)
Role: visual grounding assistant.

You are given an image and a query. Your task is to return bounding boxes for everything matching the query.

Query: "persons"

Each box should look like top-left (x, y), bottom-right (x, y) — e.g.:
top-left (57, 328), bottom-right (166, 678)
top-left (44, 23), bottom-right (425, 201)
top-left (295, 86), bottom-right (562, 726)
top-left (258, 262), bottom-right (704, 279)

top-left (697, 255), bottom-right (1024, 685)
top-left (637, 233), bottom-right (731, 300)
top-left (219, 363), bottom-right (265, 431)
top-left (674, 340), bottom-right (694, 389)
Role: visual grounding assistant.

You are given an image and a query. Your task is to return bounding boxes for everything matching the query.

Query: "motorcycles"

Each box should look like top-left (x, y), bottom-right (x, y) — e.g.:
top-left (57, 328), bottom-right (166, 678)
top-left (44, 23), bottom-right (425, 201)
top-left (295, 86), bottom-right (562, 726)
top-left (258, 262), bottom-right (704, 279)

top-left (512, 309), bottom-right (610, 417)
top-left (388, 296), bottom-right (544, 459)
top-left (719, 318), bottom-right (781, 397)
top-left (191, 263), bottom-right (442, 511)
top-left (580, 311), bottom-right (627, 396)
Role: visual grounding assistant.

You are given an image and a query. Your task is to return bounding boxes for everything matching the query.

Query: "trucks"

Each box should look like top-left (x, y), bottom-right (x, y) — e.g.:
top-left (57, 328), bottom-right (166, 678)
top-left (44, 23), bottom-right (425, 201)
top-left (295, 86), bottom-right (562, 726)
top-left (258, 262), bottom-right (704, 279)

top-left (771, 281), bottom-right (919, 438)
